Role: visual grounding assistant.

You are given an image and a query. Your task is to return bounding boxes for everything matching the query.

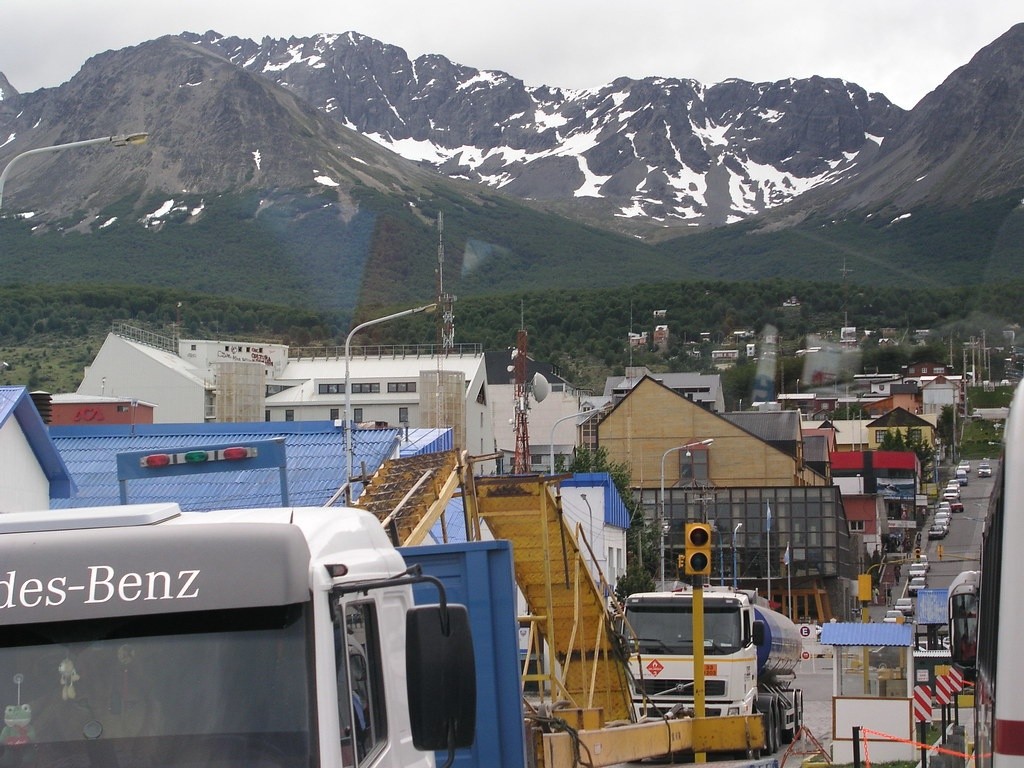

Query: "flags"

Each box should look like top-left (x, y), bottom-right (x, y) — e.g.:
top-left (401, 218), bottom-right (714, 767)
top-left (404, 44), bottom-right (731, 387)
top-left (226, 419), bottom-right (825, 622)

top-left (784, 548), bottom-right (789, 564)
top-left (767, 508), bottom-right (771, 530)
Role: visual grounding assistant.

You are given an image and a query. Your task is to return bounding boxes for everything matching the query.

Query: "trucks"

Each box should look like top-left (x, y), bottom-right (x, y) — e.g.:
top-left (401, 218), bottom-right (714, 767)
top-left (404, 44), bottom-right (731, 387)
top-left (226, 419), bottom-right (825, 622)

top-left (2, 446), bottom-right (769, 768)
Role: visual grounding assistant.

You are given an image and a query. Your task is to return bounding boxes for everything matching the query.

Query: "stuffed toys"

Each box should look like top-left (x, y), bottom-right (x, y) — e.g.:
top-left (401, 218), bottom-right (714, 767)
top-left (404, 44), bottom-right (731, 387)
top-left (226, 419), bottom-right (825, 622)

top-left (58, 656), bottom-right (78, 700)
top-left (0, 703), bottom-right (36, 744)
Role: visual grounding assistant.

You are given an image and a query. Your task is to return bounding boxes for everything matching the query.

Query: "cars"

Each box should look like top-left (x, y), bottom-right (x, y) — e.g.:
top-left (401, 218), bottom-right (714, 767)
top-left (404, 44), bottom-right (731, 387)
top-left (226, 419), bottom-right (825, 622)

top-left (977, 462), bottom-right (994, 477)
top-left (930, 456), bottom-right (972, 533)
top-left (892, 597), bottom-right (916, 616)
top-left (852, 608), bottom-right (861, 619)
top-left (908, 577), bottom-right (929, 596)
top-left (914, 636), bottom-right (951, 666)
top-left (927, 524), bottom-right (946, 541)
top-left (795, 623), bottom-right (833, 660)
top-left (869, 644), bottom-right (925, 670)
top-left (1000, 379), bottom-right (1009, 387)
top-left (907, 563), bottom-right (928, 580)
top-left (911, 555), bottom-right (931, 569)
top-left (883, 609), bottom-right (903, 623)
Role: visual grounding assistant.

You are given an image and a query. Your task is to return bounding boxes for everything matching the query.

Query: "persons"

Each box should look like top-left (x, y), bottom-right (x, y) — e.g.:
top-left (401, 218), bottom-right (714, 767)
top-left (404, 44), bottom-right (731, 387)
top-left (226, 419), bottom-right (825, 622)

top-left (895, 565), bottom-right (901, 585)
top-left (872, 586), bottom-right (879, 605)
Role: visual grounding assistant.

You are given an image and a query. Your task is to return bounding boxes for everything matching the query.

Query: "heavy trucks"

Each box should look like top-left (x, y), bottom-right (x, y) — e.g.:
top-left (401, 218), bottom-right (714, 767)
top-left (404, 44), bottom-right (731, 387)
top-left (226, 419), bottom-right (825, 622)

top-left (619, 583), bottom-right (805, 759)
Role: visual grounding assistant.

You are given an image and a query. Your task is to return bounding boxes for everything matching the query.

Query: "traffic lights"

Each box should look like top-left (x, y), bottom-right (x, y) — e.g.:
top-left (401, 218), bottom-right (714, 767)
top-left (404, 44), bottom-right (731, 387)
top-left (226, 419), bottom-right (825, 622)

top-left (915, 548), bottom-right (921, 560)
top-left (684, 522), bottom-right (712, 575)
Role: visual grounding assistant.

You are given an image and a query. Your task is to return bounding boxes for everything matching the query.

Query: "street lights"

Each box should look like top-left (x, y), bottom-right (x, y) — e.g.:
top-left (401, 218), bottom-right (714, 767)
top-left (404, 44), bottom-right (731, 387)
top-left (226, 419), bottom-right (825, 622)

top-left (733, 522), bottom-right (742, 590)
top-left (344, 301), bottom-right (439, 509)
top-left (1, 132), bottom-right (151, 202)
top-left (551, 405), bottom-right (606, 477)
top-left (660, 438), bottom-right (714, 595)
